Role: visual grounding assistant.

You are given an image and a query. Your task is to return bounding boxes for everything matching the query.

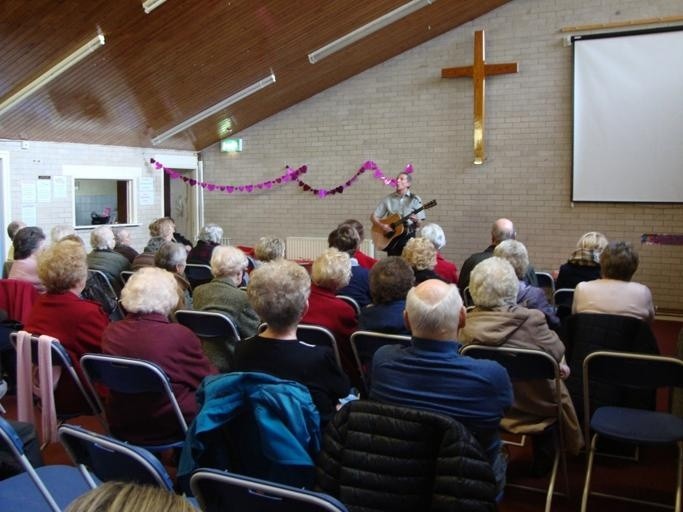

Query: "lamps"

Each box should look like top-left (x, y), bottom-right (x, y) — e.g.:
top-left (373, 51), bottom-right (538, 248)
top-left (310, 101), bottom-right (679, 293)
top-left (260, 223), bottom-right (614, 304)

top-left (9, 333), bottom-right (97, 454)
top-left (88, 269), bottom-right (126, 321)
top-left (174, 310), bottom-right (242, 342)
top-left (0, 417), bottom-right (105, 512)
top-left (336, 295), bottom-right (360, 316)
top-left (247, 256), bottom-right (255, 272)
top-left (551, 288), bottom-right (575, 314)
top-left (577, 351), bottom-right (683, 511)
top-left (120, 270), bottom-right (134, 285)
top-left (458, 345), bottom-right (563, 512)
top-left (535, 271), bottom-right (555, 312)
top-left (189, 467), bottom-right (349, 512)
top-left (80, 354), bottom-right (188, 467)
top-left (186, 264), bottom-right (215, 287)
top-left (350, 330), bottom-right (412, 395)
top-left (257, 322), bottom-right (345, 375)
top-left (56, 424), bottom-right (175, 493)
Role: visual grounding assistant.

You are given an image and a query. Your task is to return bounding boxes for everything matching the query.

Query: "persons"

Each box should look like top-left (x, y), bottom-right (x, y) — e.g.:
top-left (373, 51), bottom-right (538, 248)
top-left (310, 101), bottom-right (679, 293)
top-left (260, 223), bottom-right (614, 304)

top-left (371, 172), bottom-right (425, 258)
top-left (62, 480), bottom-right (202, 512)
top-left (358, 256), bottom-right (417, 381)
top-left (144, 217), bottom-right (174, 252)
top-left (230, 259), bottom-right (347, 437)
top-left (2, 221), bottom-right (26, 280)
top-left (101, 266), bottom-right (222, 463)
top-left (368, 280), bottom-right (513, 504)
top-left (21, 240), bottom-right (111, 412)
top-left (570, 240), bottom-right (655, 330)
top-left (457, 256), bottom-right (596, 499)
top-left (458, 218), bottom-right (538, 308)
top-left (555, 231), bottom-right (610, 323)
top-left (8, 226), bottom-right (48, 298)
top-left (492, 239), bottom-right (563, 332)
top-left (168, 218), bottom-right (193, 257)
top-left (193, 244), bottom-right (262, 341)
top-left (300, 247), bottom-right (358, 374)
top-left (187, 222), bottom-right (225, 265)
top-left (402, 238), bottom-right (445, 285)
top-left (155, 241), bottom-right (194, 310)
top-left (113, 227), bottom-right (139, 264)
top-left (415, 222), bottom-right (459, 284)
top-left (254, 236), bottom-right (284, 267)
top-left (340, 219), bottom-right (380, 269)
top-left (86, 226), bottom-right (131, 300)
top-left (329, 225), bottom-right (373, 310)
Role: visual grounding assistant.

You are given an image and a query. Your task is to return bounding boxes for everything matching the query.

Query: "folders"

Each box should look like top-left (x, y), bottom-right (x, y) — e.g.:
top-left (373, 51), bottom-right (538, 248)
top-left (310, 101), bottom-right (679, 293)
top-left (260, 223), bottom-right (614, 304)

top-left (286, 237), bottom-right (374, 265)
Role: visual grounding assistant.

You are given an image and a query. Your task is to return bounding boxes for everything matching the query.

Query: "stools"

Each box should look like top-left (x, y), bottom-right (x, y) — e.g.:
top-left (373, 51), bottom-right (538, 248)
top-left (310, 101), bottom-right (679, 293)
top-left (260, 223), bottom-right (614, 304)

top-left (405, 226), bottom-right (416, 234)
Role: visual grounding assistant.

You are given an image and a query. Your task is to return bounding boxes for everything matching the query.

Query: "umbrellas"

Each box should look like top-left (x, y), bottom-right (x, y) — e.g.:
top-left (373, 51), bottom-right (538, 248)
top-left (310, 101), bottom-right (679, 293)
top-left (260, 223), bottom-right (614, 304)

top-left (531, 455), bottom-right (554, 479)
top-left (575, 452), bottom-right (588, 467)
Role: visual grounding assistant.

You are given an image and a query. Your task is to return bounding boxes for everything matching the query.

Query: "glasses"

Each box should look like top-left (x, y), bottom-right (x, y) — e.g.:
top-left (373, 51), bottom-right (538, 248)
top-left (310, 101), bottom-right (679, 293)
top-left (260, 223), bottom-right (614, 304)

top-left (151, 75), bottom-right (277, 145)
top-left (220, 138), bottom-right (243, 152)
top-left (308, 0), bottom-right (432, 65)
top-left (142, 0), bottom-right (166, 14)
top-left (0, 35), bottom-right (105, 115)
